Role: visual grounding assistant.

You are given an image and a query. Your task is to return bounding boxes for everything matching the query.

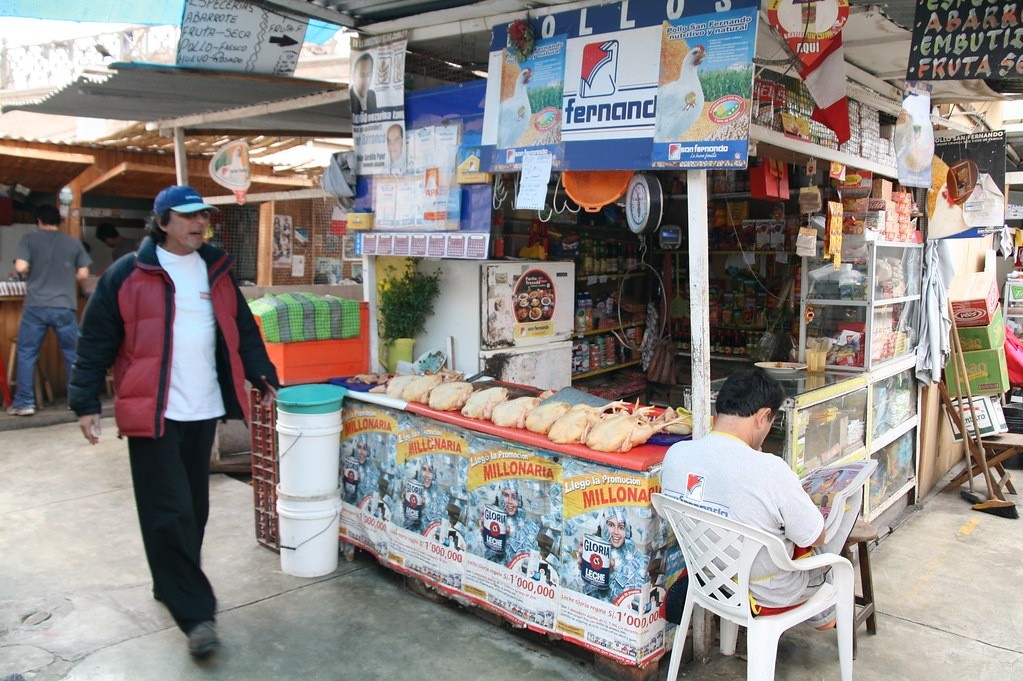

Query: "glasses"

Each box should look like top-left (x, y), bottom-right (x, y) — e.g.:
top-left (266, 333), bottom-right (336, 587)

top-left (172, 211), bottom-right (211, 219)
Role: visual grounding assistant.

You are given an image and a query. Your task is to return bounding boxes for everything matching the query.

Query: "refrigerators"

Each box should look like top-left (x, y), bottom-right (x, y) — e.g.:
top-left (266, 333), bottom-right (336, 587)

top-left (416, 256), bottom-right (576, 393)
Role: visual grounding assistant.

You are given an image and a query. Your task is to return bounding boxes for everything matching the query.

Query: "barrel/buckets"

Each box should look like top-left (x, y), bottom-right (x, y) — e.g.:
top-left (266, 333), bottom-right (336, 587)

top-left (274, 407), bottom-right (344, 497)
top-left (275, 483), bottom-right (343, 578)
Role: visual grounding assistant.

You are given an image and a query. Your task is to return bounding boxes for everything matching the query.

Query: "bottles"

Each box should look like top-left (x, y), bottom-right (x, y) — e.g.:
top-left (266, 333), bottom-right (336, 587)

top-left (490, 215), bottom-right (504, 260)
top-left (572, 233), bottom-right (763, 376)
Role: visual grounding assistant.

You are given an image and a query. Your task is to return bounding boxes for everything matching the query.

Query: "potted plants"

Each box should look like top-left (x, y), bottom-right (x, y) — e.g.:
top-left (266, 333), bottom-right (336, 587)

top-left (379, 255), bottom-right (445, 376)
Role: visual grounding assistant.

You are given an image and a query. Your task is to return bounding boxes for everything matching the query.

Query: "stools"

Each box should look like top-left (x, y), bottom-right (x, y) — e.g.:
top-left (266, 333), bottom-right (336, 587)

top-left (3, 336), bottom-right (53, 409)
top-left (833, 520), bottom-right (879, 661)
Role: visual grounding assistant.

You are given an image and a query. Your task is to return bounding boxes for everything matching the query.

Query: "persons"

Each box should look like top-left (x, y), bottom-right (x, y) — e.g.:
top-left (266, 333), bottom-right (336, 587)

top-left (387, 124), bottom-right (404, 173)
top-left (96, 223), bottom-right (139, 262)
top-left (7, 207), bottom-right (92, 414)
top-left (69, 187), bottom-right (279, 658)
top-left (473, 479), bottom-right (541, 566)
top-left (660, 367), bottom-right (837, 630)
top-left (349, 53), bottom-right (377, 113)
top-left (402, 454), bottom-right (449, 534)
top-left (577, 506), bottom-right (641, 606)
top-left (803, 471), bottom-right (843, 503)
top-left (342, 433), bottom-right (379, 507)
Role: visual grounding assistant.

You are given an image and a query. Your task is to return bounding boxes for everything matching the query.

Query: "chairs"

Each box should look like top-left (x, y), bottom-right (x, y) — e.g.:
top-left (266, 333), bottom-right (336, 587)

top-left (938, 381), bottom-right (1023, 501)
top-left (649, 494), bottom-right (854, 681)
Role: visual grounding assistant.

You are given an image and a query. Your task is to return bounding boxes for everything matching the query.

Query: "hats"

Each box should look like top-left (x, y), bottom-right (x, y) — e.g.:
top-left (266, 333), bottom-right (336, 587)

top-left (154, 184), bottom-right (220, 215)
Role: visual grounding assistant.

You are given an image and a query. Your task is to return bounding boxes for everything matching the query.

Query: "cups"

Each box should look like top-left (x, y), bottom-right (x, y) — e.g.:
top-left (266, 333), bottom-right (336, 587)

top-left (805, 348), bottom-right (827, 373)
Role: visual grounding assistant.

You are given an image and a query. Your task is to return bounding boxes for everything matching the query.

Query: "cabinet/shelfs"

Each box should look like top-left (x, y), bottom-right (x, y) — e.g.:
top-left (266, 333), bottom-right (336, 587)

top-left (494, 184), bottom-right (654, 403)
top-left (1003, 280), bottom-right (1023, 350)
top-left (658, 191), bottom-right (806, 362)
top-left (798, 235), bottom-right (924, 377)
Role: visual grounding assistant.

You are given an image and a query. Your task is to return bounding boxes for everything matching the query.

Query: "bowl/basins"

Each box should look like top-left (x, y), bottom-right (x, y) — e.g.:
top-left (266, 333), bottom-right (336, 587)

top-left (518, 291), bottom-right (551, 320)
top-left (273, 382), bottom-right (349, 415)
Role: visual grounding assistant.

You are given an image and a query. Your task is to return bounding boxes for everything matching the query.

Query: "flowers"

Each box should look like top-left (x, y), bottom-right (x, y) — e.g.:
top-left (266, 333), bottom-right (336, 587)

top-left (506, 10), bottom-right (535, 61)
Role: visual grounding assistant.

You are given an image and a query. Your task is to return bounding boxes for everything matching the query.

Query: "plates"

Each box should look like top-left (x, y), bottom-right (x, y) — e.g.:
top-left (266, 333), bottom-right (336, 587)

top-left (754, 362), bottom-right (808, 374)
top-left (412, 348), bottom-right (447, 376)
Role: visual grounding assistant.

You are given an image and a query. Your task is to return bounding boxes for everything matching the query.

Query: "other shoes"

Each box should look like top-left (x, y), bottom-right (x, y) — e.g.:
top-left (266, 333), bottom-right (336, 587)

top-left (187, 619), bottom-right (220, 655)
top-left (6, 406), bottom-right (35, 417)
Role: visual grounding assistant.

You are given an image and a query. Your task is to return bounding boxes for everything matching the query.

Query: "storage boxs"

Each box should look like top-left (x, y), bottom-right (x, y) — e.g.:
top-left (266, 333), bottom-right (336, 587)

top-left (786, 79), bottom-right (898, 167)
top-left (710, 266), bottom-right (779, 331)
top-left (252, 299), bottom-right (368, 553)
top-left (710, 156), bottom-right (923, 245)
top-left (834, 321), bottom-right (906, 369)
top-left (942, 258), bottom-right (1010, 443)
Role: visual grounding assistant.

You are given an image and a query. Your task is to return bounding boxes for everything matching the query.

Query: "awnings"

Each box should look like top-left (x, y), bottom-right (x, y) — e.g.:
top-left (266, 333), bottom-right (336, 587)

top-left (1, 61), bottom-right (352, 138)
